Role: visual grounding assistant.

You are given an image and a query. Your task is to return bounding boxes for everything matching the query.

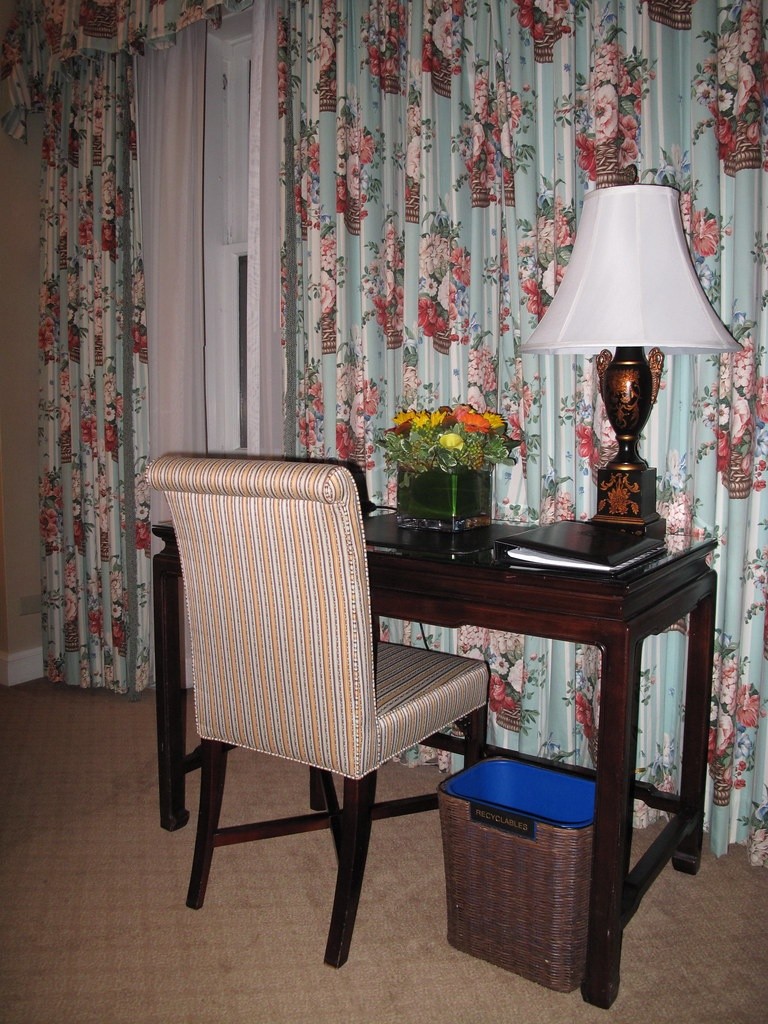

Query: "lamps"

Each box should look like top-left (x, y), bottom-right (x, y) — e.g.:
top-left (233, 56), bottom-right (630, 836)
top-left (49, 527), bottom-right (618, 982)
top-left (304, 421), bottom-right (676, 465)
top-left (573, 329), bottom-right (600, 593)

top-left (516, 183), bottom-right (745, 536)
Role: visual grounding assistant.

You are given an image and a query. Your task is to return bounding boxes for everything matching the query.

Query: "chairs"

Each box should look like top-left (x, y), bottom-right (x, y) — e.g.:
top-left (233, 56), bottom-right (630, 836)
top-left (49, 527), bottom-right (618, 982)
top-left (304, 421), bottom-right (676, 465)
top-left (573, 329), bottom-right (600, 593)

top-left (144, 453), bottom-right (491, 966)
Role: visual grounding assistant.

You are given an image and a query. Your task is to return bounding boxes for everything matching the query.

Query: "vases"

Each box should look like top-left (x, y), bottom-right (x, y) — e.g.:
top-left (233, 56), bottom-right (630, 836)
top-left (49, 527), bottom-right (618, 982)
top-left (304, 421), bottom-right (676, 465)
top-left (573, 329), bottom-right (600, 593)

top-left (396, 467), bottom-right (492, 531)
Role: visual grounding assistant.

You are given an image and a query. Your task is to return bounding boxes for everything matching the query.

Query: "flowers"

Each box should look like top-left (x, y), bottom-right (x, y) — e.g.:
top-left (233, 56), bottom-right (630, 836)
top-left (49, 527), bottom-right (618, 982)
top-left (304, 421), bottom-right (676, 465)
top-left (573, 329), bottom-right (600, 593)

top-left (370, 402), bottom-right (521, 477)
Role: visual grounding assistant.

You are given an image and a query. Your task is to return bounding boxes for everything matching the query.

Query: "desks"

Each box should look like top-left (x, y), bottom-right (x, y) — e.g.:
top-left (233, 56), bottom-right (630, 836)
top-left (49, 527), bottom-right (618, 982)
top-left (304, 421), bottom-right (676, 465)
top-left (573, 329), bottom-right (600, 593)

top-left (153, 508), bottom-right (721, 1012)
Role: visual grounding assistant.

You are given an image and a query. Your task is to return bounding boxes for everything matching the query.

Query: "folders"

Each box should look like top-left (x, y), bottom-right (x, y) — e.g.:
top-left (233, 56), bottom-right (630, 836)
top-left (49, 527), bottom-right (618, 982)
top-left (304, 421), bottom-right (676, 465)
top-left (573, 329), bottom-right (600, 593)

top-left (490, 519), bottom-right (667, 578)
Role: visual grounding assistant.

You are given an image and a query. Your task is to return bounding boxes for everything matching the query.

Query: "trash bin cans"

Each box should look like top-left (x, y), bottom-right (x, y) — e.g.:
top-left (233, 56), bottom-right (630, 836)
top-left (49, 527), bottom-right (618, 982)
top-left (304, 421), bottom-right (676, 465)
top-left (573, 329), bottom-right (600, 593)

top-left (435, 754), bottom-right (598, 994)
